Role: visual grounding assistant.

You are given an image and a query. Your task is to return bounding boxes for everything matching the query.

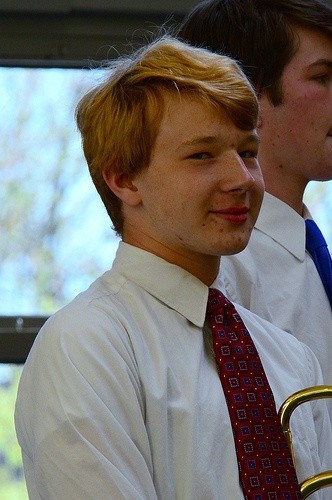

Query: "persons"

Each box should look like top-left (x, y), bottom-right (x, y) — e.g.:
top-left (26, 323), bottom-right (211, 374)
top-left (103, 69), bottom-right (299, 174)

top-left (172, 0), bottom-right (332, 421)
top-left (14, 37), bottom-right (332, 500)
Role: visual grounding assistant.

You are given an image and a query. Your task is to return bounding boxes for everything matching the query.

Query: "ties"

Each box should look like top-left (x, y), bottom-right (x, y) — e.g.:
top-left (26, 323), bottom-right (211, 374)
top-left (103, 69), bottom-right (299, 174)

top-left (207, 288), bottom-right (303, 500)
top-left (305, 219), bottom-right (332, 312)
